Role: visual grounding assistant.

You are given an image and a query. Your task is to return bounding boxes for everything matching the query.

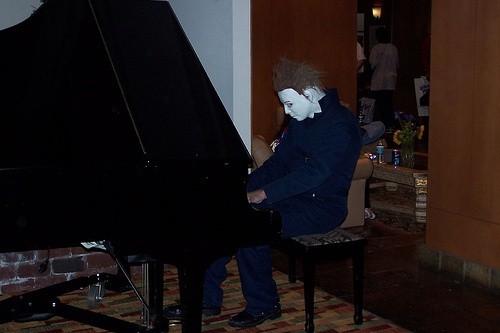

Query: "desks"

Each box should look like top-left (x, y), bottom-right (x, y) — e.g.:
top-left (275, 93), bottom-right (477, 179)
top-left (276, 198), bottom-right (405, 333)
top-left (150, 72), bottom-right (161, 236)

top-left (369, 160), bottom-right (427, 224)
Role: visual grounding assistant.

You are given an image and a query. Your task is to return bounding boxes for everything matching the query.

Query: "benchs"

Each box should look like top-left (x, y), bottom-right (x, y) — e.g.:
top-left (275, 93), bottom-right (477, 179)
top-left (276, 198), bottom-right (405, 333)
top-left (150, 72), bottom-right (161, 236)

top-left (276, 226), bottom-right (368, 333)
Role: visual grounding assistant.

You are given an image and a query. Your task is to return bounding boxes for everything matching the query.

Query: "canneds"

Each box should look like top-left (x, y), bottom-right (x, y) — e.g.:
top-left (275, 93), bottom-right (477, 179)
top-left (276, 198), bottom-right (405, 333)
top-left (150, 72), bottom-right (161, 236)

top-left (392, 149), bottom-right (401, 166)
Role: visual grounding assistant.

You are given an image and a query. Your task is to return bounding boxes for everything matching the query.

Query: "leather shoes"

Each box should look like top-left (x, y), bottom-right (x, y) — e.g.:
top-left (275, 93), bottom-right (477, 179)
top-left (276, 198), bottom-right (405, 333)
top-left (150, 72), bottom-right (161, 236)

top-left (164, 300), bottom-right (221, 320)
top-left (229, 300), bottom-right (282, 328)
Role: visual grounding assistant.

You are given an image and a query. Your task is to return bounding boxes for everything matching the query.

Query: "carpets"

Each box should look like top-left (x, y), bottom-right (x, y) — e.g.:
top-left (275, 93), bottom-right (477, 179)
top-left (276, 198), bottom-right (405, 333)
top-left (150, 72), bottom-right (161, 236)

top-left (0, 259), bottom-right (407, 333)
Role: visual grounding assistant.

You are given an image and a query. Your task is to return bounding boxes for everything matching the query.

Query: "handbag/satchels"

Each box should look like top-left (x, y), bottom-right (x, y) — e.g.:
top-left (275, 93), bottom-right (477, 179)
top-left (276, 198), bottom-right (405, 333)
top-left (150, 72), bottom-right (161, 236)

top-left (360, 97), bottom-right (376, 123)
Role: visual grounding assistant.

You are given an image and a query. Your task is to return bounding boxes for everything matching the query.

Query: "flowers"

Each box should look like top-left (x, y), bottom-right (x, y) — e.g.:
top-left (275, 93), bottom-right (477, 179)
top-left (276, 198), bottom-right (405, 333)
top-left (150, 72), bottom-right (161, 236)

top-left (389, 111), bottom-right (425, 145)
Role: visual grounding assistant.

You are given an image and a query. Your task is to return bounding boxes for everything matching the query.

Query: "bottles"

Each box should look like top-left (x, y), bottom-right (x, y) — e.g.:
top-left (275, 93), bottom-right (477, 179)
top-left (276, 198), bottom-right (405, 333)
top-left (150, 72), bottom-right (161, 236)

top-left (376, 139), bottom-right (384, 164)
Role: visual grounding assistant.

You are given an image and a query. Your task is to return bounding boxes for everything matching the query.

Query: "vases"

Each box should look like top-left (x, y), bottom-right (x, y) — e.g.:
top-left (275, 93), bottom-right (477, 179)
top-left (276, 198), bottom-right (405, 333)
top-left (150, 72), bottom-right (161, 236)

top-left (399, 141), bottom-right (415, 170)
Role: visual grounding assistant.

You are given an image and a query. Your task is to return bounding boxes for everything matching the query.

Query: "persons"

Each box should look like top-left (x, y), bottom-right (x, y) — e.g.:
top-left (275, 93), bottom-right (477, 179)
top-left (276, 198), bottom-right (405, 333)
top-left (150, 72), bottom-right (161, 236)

top-left (408, 31), bottom-right (431, 149)
top-left (269, 114), bottom-right (377, 220)
top-left (369, 27), bottom-right (399, 125)
top-left (357, 42), bottom-right (367, 118)
top-left (163, 59), bottom-right (363, 328)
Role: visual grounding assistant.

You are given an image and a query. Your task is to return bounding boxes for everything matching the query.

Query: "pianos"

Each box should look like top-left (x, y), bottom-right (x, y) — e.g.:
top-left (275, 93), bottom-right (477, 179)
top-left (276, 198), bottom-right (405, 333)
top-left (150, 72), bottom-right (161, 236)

top-left (0, 0), bottom-right (282, 333)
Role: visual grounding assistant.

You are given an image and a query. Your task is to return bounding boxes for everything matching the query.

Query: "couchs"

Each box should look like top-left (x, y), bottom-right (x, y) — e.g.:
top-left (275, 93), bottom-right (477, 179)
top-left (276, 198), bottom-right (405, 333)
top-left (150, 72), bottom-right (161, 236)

top-left (251, 134), bottom-right (374, 228)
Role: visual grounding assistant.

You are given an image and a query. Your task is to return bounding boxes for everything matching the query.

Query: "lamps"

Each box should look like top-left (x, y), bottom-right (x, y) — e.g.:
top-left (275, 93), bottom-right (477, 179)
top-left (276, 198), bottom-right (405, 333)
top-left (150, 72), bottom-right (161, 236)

top-left (372, 7), bottom-right (381, 19)
